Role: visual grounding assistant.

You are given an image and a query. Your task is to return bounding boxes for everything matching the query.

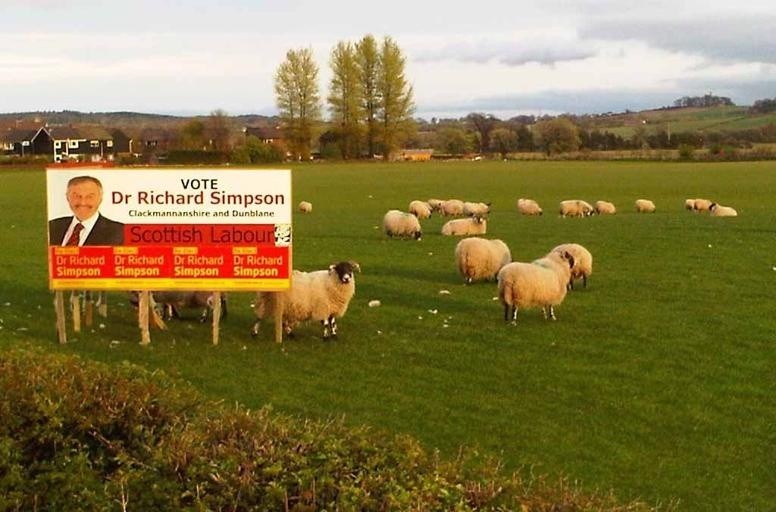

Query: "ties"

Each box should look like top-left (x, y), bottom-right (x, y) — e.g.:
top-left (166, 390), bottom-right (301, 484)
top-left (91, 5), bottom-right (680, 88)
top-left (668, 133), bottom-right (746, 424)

top-left (65, 224), bottom-right (84, 246)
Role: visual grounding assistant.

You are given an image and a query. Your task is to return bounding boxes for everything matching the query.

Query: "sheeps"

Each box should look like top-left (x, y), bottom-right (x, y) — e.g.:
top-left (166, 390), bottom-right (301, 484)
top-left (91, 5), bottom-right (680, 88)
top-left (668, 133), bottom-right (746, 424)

top-left (635, 198), bottom-right (658, 213)
top-left (545, 239), bottom-right (594, 291)
top-left (382, 197), bottom-right (492, 241)
top-left (516, 197), bottom-right (544, 218)
top-left (298, 199), bottom-right (313, 213)
top-left (250, 258), bottom-right (360, 341)
top-left (495, 248), bottom-right (575, 328)
top-left (684, 198), bottom-right (737, 217)
top-left (126, 291), bottom-right (229, 325)
top-left (558, 199), bottom-right (617, 218)
top-left (453, 236), bottom-right (512, 288)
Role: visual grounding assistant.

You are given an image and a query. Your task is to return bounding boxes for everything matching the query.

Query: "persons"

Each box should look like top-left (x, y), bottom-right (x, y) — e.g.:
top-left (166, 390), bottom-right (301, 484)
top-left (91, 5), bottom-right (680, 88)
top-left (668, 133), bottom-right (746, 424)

top-left (49, 175), bottom-right (124, 247)
